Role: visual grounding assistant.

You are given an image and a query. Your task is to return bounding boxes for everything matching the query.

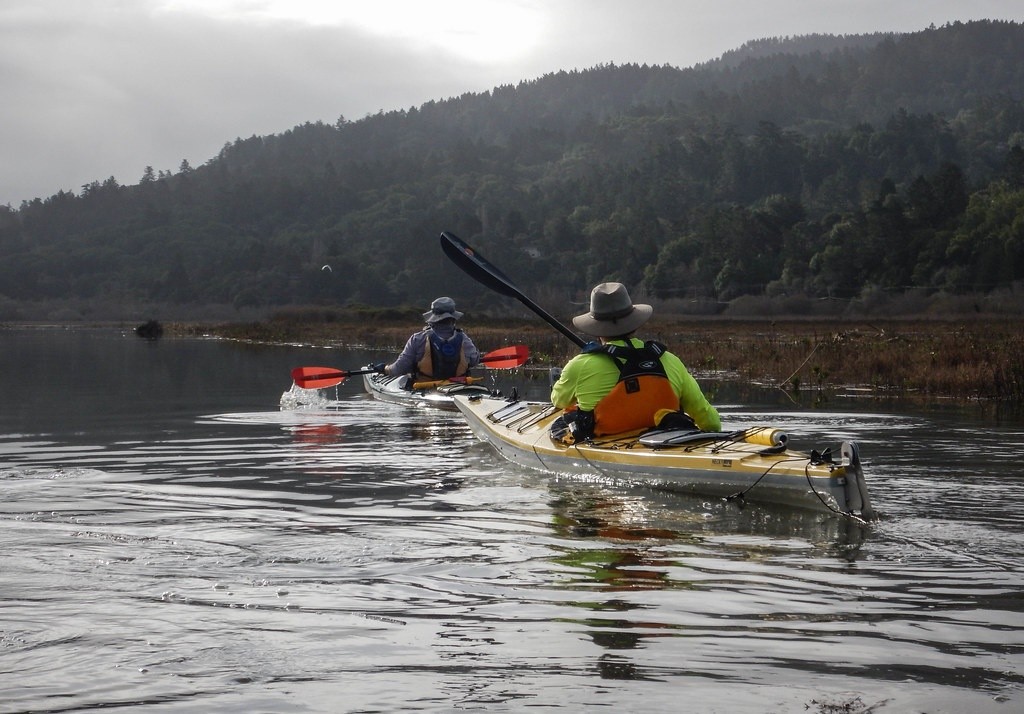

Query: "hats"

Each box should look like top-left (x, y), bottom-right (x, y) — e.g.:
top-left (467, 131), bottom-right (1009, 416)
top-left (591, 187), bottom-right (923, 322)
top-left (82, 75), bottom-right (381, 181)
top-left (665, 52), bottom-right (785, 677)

top-left (422, 297), bottom-right (464, 323)
top-left (572, 282), bottom-right (653, 337)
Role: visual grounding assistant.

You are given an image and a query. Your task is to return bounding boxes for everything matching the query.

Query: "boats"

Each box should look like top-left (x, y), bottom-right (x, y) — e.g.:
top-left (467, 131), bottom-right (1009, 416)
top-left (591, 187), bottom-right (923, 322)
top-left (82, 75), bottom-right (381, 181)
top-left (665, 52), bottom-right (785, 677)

top-left (452, 384), bottom-right (873, 520)
top-left (359, 362), bottom-right (519, 414)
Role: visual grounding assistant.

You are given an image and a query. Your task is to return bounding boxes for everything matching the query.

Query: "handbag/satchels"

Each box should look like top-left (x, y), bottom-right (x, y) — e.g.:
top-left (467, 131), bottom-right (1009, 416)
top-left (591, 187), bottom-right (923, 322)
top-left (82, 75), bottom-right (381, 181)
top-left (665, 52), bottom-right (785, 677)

top-left (549, 409), bottom-right (597, 444)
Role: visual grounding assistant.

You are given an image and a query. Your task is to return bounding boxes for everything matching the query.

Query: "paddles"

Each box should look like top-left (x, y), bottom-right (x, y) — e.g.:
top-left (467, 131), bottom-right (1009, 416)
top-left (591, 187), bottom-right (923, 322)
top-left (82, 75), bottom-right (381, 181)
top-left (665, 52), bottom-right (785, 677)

top-left (288, 344), bottom-right (530, 390)
top-left (436, 228), bottom-right (591, 351)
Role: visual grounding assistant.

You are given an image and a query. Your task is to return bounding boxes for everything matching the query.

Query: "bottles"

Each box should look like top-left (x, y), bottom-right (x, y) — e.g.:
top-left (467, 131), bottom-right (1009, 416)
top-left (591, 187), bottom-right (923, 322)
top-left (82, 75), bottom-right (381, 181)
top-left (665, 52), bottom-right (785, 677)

top-left (567, 421), bottom-right (577, 432)
top-left (744, 426), bottom-right (791, 448)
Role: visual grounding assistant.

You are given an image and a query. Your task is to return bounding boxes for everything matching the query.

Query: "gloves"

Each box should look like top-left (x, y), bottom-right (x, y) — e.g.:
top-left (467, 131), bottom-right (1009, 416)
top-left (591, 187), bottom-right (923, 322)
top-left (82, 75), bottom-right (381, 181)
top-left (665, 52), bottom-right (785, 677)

top-left (549, 367), bottom-right (562, 385)
top-left (374, 363), bottom-right (386, 374)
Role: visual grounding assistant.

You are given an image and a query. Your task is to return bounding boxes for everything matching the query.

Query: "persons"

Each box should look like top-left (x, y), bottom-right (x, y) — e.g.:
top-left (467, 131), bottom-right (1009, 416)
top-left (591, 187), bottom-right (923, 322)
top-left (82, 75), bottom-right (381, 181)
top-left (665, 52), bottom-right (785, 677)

top-left (373, 297), bottom-right (480, 381)
top-left (550, 281), bottom-right (722, 433)
